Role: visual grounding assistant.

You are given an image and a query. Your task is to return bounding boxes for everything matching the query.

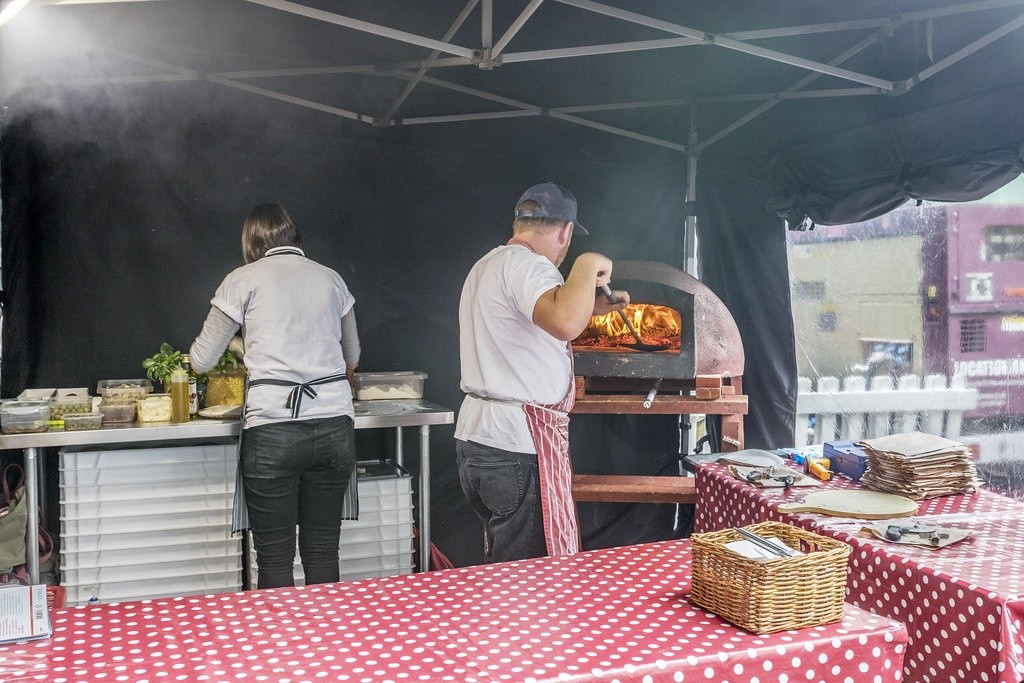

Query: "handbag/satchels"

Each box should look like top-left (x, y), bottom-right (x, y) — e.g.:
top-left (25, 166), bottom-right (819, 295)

top-left (0, 463), bottom-right (53, 582)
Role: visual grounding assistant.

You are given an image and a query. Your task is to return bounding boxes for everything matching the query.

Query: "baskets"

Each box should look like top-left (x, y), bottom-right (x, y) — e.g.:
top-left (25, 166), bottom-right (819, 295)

top-left (690, 520), bottom-right (852, 634)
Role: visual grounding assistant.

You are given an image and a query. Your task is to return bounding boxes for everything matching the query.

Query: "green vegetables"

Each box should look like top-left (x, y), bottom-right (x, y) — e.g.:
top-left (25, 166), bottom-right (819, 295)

top-left (143, 342), bottom-right (238, 384)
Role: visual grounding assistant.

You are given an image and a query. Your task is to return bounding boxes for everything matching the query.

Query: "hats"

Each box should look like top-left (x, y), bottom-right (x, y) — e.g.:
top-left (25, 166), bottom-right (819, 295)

top-left (515, 181), bottom-right (589, 235)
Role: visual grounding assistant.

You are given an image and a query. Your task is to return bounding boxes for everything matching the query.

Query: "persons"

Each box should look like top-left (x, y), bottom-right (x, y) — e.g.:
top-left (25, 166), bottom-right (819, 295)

top-left (453, 182), bottom-right (631, 569)
top-left (188, 200), bottom-right (361, 592)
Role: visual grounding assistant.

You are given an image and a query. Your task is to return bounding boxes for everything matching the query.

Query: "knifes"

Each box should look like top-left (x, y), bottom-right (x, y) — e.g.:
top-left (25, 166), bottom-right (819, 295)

top-left (886, 525), bottom-right (937, 542)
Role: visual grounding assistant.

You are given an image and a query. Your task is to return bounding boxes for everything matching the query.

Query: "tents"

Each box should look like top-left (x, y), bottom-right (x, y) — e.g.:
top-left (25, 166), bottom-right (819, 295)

top-left (0, 0), bottom-right (1023, 683)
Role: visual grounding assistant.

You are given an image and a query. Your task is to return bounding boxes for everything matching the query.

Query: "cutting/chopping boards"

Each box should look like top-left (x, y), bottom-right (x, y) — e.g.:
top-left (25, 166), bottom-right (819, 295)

top-left (777, 490), bottom-right (918, 520)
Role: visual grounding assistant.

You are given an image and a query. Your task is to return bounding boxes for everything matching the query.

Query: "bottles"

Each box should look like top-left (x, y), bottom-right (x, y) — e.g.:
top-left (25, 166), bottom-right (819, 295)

top-left (171, 361), bottom-right (189, 423)
top-left (181, 357), bottom-right (198, 421)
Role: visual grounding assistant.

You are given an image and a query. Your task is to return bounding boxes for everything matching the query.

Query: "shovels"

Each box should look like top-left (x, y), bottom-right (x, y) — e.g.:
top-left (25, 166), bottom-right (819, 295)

top-left (597, 271), bottom-right (669, 352)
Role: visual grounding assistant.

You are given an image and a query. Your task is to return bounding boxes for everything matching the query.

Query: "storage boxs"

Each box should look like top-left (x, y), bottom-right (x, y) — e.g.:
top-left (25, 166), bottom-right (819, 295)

top-left (57, 437), bottom-right (415, 610)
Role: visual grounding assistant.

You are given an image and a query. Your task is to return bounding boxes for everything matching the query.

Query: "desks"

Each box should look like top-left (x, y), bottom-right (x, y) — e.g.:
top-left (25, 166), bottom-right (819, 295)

top-left (0, 538), bottom-right (907, 683)
top-left (682, 445), bottom-right (1024, 683)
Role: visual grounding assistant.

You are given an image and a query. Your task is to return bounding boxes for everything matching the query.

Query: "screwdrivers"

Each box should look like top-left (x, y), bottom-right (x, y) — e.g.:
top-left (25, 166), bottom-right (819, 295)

top-left (777, 448), bottom-right (805, 464)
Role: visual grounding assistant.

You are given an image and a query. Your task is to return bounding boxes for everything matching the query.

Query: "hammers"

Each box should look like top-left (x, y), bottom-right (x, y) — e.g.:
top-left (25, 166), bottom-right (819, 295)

top-left (919, 532), bottom-right (949, 544)
